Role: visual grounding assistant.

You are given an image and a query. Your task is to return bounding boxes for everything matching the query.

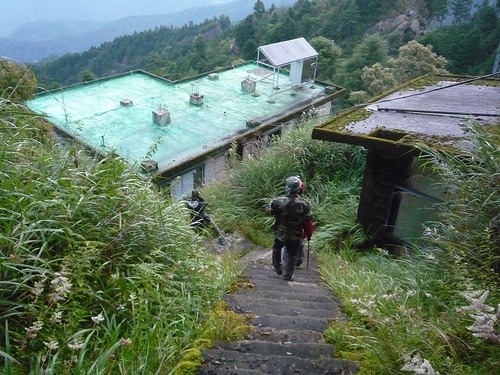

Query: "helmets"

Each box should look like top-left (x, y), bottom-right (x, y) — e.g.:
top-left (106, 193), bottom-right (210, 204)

top-left (286, 177), bottom-right (301, 192)
top-left (300, 181), bottom-right (304, 190)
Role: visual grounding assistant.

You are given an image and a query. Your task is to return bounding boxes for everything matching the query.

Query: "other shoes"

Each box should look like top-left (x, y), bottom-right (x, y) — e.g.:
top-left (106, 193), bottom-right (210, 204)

top-left (271, 266), bottom-right (282, 275)
top-left (285, 272), bottom-right (294, 280)
top-left (294, 262), bottom-right (303, 266)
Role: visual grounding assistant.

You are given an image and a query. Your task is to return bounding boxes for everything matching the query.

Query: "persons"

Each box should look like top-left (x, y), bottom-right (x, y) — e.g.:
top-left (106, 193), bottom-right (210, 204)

top-left (185, 190), bottom-right (210, 238)
top-left (268, 176), bottom-right (313, 280)
top-left (283, 182), bottom-right (312, 267)
